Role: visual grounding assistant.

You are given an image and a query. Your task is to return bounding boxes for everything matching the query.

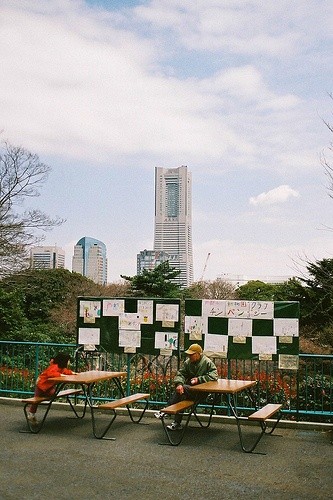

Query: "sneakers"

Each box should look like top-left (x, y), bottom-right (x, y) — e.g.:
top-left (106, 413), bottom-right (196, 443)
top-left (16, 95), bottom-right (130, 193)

top-left (154, 411), bottom-right (171, 419)
top-left (166, 421), bottom-right (182, 430)
top-left (26, 416), bottom-right (38, 425)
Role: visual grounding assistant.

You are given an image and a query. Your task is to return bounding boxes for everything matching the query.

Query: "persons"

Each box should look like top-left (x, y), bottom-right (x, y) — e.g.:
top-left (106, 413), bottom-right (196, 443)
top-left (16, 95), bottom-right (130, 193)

top-left (26, 351), bottom-right (78, 426)
top-left (155, 342), bottom-right (219, 431)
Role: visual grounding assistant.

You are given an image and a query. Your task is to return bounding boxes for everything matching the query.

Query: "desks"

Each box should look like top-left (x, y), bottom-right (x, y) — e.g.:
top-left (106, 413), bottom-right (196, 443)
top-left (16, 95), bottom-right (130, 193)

top-left (189, 379), bottom-right (257, 395)
top-left (47, 370), bottom-right (127, 384)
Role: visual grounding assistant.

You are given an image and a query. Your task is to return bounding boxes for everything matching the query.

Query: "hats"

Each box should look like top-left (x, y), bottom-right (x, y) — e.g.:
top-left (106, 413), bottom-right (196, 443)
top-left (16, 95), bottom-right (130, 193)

top-left (184, 344), bottom-right (202, 354)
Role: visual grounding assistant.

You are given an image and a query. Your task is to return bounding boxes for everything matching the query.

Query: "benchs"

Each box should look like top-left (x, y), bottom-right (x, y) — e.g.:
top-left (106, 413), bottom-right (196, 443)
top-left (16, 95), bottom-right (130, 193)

top-left (236, 403), bottom-right (284, 453)
top-left (19, 388), bottom-right (87, 433)
top-left (160, 399), bottom-right (215, 446)
top-left (90, 392), bottom-right (151, 439)
